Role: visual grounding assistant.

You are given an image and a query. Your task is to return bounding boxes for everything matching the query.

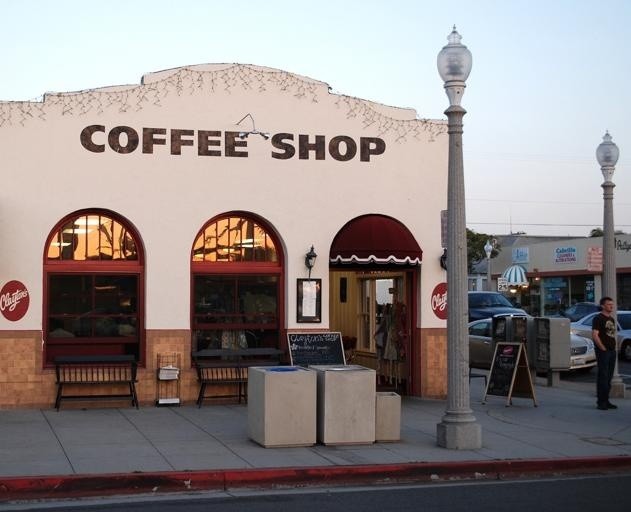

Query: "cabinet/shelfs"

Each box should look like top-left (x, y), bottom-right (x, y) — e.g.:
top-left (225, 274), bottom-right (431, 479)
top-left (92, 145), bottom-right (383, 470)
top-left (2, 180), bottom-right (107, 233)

top-left (154, 350), bottom-right (184, 408)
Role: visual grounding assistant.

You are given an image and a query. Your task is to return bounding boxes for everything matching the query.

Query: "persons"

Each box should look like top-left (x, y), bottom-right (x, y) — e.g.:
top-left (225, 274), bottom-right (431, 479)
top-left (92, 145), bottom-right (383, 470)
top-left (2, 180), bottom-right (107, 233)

top-left (592, 296), bottom-right (617, 411)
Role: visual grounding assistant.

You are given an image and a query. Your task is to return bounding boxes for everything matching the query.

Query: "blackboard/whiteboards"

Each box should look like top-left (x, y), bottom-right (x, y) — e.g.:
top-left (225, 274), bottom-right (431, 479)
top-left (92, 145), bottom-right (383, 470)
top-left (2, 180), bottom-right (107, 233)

top-left (486, 342), bottom-right (523, 397)
top-left (287, 332), bottom-right (347, 366)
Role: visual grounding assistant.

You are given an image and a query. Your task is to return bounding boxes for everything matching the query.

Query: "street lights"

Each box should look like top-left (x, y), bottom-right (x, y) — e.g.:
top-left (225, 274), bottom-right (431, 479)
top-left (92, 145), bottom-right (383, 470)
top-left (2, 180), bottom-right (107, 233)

top-left (594, 130), bottom-right (626, 400)
top-left (483, 237), bottom-right (494, 290)
top-left (435, 23), bottom-right (482, 452)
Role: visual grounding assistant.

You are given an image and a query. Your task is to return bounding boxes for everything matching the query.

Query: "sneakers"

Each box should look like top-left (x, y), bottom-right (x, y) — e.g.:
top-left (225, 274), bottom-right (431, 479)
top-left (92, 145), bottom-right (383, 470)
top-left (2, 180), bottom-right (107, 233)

top-left (595, 399), bottom-right (618, 412)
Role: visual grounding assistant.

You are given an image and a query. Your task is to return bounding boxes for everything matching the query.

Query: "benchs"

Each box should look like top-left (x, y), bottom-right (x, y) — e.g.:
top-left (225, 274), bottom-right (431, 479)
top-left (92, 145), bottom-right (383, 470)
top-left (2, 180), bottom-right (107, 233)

top-left (188, 344), bottom-right (291, 408)
top-left (49, 353), bottom-right (143, 410)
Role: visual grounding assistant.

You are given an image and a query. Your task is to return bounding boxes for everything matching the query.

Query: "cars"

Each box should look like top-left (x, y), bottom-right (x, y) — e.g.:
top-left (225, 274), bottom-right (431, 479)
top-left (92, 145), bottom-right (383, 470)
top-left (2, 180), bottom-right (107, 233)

top-left (48, 308), bottom-right (136, 340)
top-left (468, 288), bottom-right (631, 377)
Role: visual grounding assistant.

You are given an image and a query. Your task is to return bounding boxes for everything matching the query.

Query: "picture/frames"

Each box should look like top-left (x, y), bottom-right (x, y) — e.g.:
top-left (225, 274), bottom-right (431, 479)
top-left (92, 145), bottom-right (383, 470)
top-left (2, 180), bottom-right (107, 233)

top-left (295, 277), bottom-right (322, 323)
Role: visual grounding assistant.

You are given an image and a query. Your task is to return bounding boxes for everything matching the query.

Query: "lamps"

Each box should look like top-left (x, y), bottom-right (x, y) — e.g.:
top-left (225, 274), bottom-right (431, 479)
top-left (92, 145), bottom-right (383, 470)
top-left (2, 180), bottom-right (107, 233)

top-left (441, 247), bottom-right (449, 273)
top-left (304, 244), bottom-right (318, 276)
top-left (236, 113), bottom-right (271, 143)
